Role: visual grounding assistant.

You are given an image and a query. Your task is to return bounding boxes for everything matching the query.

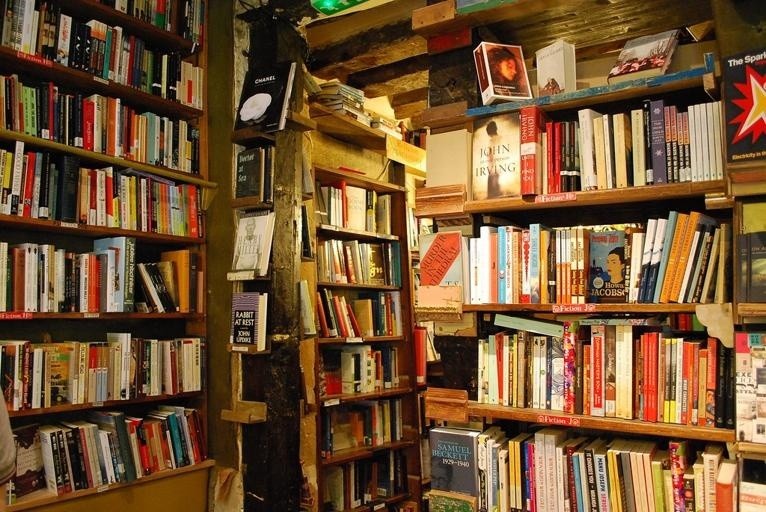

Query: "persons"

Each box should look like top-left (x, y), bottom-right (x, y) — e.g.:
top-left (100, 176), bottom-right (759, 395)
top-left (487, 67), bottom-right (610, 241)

top-left (490, 47), bottom-right (522, 95)
top-left (487, 122), bottom-right (501, 197)
top-left (232, 219), bottom-right (261, 271)
top-left (0, 387), bottom-right (17, 512)
top-left (597, 247), bottom-right (624, 302)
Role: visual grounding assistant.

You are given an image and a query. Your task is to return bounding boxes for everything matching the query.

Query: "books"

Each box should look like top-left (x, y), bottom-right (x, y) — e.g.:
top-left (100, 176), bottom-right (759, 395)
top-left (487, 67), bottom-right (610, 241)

top-left (586, 230), bottom-right (624, 302)
top-left (11, 405), bottom-right (209, 498)
top-left (0, 236), bottom-right (204, 313)
top-left (429, 416), bottom-right (739, 511)
top-left (0, 141), bottom-right (202, 238)
top-left (0, 333), bottom-right (209, 412)
top-left (321, 397), bottom-right (403, 460)
top-left (235, 62), bottom-right (297, 133)
top-left (479, 314), bottom-right (734, 430)
top-left (300, 235), bottom-right (402, 287)
top-left (734, 331), bottom-right (766, 446)
top-left (472, 41), bottom-right (534, 106)
top-left (0, 74), bottom-right (200, 175)
top-left (608, 28), bottom-right (681, 85)
top-left (519, 100), bottom-right (724, 195)
top-left (1, 0), bottom-right (204, 111)
top-left (720, 46), bottom-right (766, 170)
top-left (535, 39), bottom-right (577, 98)
top-left (115, 0), bottom-right (204, 47)
top-left (419, 224), bottom-right (589, 305)
top-left (232, 213), bottom-right (275, 277)
top-left (306, 80), bottom-right (426, 149)
top-left (236, 145), bottom-right (276, 203)
top-left (324, 446), bottom-right (409, 512)
top-left (325, 345), bottom-right (400, 394)
top-left (301, 184), bottom-right (392, 235)
top-left (623, 202), bottom-right (766, 304)
top-left (230, 292), bottom-right (268, 352)
top-left (300, 288), bottom-right (404, 337)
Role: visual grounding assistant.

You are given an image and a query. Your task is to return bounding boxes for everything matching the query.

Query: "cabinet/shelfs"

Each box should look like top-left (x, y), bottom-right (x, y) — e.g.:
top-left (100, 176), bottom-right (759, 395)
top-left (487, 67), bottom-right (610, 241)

top-left (414, 181), bottom-right (477, 512)
top-left (219, 17), bottom-right (419, 512)
top-left (464, 51), bottom-right (736, 512)
top-left (727, 160), bottom-right (766, 512)
top-left (0, 1), bottom-right (217, 512)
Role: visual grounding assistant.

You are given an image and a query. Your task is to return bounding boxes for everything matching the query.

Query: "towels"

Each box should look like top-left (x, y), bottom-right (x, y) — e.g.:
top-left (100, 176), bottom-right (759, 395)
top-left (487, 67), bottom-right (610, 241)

top-left (208, 466), bottom-right (245, 512)
